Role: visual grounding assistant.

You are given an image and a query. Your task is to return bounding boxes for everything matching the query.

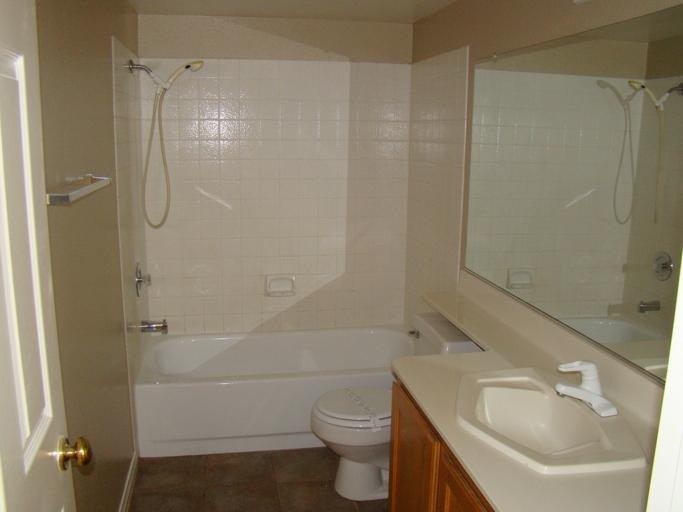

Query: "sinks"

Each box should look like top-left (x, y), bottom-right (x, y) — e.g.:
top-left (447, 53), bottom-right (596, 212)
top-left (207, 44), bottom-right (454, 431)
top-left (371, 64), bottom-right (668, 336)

top-left (632, 356), bottom-right (666, 381)
top-left (454, 366), bottom-right (649, 475)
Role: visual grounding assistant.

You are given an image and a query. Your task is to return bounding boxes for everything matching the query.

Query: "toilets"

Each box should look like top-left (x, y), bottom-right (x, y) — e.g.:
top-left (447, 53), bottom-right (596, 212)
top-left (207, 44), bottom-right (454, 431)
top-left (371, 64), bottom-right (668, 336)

top-left (309, 311), bottom-right (484, 502)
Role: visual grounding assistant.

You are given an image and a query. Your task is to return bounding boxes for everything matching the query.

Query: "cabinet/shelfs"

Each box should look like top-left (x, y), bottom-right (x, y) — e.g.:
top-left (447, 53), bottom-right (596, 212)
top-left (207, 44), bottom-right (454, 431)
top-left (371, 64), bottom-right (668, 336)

top-left (389, 345), bottom-right (683, 511)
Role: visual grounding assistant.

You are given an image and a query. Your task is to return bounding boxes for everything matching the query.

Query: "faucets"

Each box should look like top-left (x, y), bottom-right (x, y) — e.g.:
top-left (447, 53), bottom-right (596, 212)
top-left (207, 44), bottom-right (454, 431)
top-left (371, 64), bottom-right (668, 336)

top-left (141, 319), bottom-right (169, 334)
top-left (125, 321), bottom-right (141, 334)
top-left (636, 300), bottom-right (662, 312)
top-left (553, 360), bottom-right (617, 417)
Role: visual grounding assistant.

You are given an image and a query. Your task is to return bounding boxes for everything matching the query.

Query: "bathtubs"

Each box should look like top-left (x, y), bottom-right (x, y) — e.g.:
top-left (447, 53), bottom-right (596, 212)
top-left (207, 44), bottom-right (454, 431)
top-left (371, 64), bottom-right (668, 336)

top-left (560, 316), bottom-right (665, 340)
top-left (133, 324), bottom-right (440, 460)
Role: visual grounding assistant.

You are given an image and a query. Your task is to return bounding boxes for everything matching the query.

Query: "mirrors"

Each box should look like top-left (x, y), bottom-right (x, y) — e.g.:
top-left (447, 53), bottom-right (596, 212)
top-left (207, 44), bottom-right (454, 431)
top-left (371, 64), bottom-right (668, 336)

top-left (459, 0), bottom-right (683, 386)
